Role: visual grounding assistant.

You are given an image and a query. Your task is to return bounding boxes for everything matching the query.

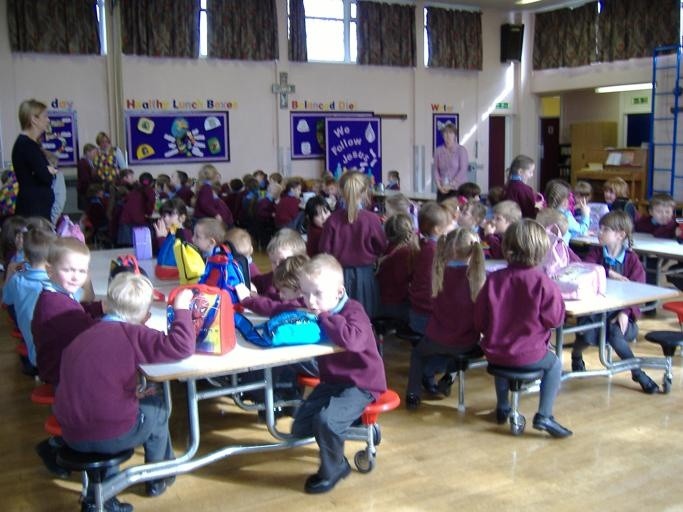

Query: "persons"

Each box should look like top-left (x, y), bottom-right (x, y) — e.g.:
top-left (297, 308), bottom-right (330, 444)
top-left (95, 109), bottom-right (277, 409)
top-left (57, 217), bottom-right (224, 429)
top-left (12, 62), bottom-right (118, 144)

top-left (93, 131), bottom-right (125, 182)
top-left (74, 144), bottom-right (99, 211)
top-left (430, 122), bottom-right (468, 201)
top-left (381, 156), bottom-right (683, 436)
top-left (0, 155), bottom-right (390, 512)
top-left (9, 97), bottom-right (56, 225)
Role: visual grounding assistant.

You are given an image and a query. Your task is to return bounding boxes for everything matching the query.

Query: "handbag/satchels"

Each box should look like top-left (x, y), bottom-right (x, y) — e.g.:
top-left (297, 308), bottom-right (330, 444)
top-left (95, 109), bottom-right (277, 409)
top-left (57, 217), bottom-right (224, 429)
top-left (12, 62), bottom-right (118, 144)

top-left (131, 226), bottom-right (153, 260)
top-left (165, 284), bottom-right (236, 356)
top-left (107, 254), bottom-right (148, 286)
top-left (233, 310), bottom-right (321, 348)
top-left (172, 238), bottom-right (205, 285)
top-left (155, 222), bottom-right (178, 280)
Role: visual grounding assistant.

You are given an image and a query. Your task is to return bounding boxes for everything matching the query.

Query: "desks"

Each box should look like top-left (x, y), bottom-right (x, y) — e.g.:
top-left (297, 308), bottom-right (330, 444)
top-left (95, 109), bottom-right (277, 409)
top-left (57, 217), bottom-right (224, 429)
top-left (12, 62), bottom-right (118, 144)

top-left (483, 256), bottom-right (680, 435)
top-left (570, 233), bottom-right (683, 288)
top-left (90, 247), bottom-right (380, 512)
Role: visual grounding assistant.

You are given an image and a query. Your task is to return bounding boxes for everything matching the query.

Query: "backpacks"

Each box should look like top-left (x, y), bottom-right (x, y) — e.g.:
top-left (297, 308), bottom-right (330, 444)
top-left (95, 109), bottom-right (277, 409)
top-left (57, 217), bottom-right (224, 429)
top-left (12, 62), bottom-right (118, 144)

top-left (197, 243), bottom-right (246, 313)
top-left (586, 203), bottom-right (609, 237)
top-left (549, 261), bottom-right (607, 299)
top-left (56, 214), bottom-right (85, 245)
top-left (543, 223), bottom-right (570, 279)
top-left (219, 240), bottom-right (250, 292)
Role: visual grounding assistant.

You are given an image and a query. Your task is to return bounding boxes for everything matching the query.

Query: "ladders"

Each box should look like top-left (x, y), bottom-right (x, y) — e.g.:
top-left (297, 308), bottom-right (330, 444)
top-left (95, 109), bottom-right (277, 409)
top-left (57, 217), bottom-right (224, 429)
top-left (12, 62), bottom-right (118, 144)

top-left (647, 39), bottom-right (683, 201)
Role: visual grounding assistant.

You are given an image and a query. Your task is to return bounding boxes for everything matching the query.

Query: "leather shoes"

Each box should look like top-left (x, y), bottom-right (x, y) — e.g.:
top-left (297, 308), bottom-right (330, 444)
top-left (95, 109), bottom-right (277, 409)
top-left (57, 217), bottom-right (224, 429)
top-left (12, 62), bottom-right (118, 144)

top-left (437, 373), bottom-right (453, 396)
top-left (404, 391), bottom-right (421, 409)
top-left (532, 412), bottom-right (572, 437)
top-left (144, 475), bottom-right (175, 495)
top-left (373, 422), bottom-right (381, 445)
top-left (34, 437), bottom-right (65, 479)
top-left (496, 406), bottom-right (511, 422)
top-left (631, 371), bottom-right (659, 394)
top-left (80, 495), bottom-right (132, 512)
top-left (304, 454), bottom-right (350, 493)
top-left (420, 373), bottom-right (439, 394)
top-left (571, 352), bottom-right (586, 371)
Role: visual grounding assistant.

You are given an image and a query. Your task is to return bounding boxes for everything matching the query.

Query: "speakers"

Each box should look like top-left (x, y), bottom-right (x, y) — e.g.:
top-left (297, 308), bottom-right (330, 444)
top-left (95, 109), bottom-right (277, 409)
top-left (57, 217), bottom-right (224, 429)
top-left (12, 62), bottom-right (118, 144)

top-left (500, 24), bottom-right (524, 62)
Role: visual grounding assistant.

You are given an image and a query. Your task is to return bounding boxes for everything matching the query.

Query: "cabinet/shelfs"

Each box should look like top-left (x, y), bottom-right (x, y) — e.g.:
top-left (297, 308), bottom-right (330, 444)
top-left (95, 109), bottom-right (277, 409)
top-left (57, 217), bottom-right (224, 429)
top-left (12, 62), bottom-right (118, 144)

top-left (570, 122), bottom-right (649, 208)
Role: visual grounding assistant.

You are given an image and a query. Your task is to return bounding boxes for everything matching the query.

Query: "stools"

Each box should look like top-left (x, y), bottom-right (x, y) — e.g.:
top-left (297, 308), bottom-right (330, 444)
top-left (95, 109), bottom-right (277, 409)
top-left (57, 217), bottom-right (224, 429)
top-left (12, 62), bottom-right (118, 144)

top-left (645, 331), bottom-right (683, 380)
top-left (0, 302), bottom-right (134, 512)
top-left (360, 389), bottom-right (401, 457)
top-left (297, 373), bottom-right (321, 387)
top-left (388, 328), bottom-right (544, 435)
top-left (663, 302), bottom-right (683, 332)
